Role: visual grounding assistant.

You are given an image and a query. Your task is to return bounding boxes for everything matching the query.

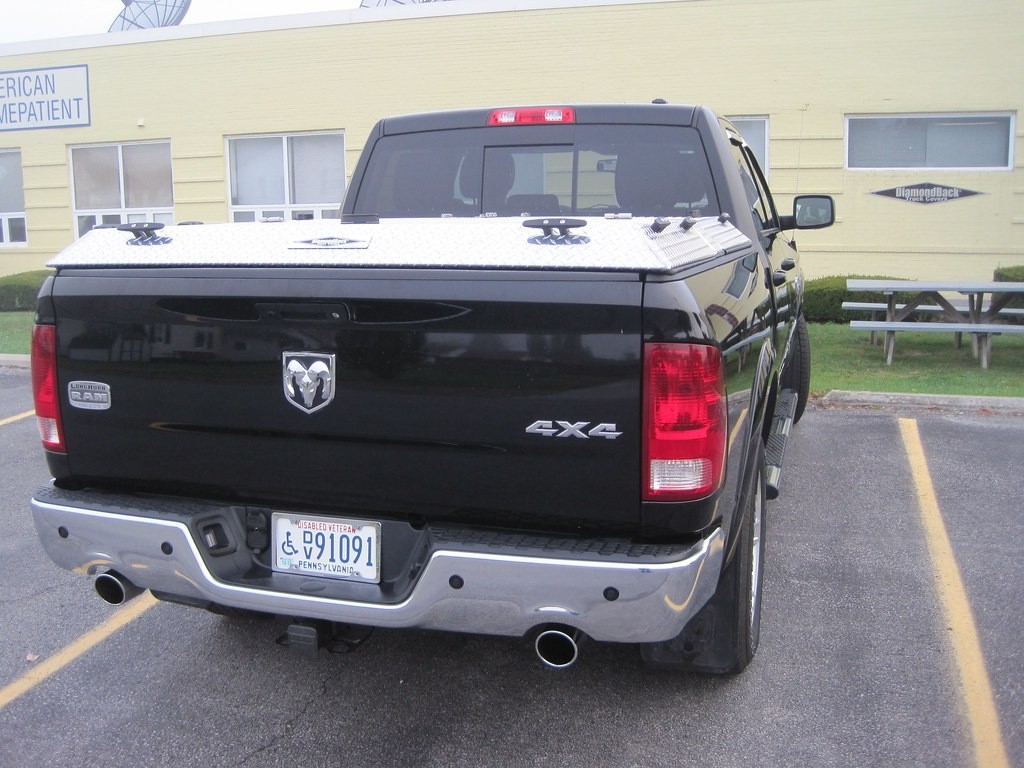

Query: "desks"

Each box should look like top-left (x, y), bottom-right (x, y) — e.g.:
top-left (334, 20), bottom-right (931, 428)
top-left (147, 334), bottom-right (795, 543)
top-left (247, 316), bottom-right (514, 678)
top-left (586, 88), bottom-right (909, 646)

top-left (844, 279), bottom-right (1024, 368)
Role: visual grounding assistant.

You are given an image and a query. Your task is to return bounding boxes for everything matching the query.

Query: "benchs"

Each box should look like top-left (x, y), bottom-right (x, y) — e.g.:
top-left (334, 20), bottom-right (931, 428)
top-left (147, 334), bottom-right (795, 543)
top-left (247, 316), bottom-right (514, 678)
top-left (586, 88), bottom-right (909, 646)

top-left (842, 302), bottom-right (1024, 371)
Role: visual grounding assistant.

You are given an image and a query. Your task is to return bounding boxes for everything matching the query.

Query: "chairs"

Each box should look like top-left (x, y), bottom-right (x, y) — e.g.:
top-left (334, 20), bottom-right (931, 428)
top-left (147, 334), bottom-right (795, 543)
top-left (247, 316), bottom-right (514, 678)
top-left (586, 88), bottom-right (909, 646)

top-left (453, 148), bottom-right (518, 215)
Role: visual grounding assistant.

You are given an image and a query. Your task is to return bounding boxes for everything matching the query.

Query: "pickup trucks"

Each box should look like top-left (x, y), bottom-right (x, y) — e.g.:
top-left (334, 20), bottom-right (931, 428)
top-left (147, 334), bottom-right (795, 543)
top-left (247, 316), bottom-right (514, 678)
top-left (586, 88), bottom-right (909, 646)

top-left (29, 97), bottom-right (836, 680)
